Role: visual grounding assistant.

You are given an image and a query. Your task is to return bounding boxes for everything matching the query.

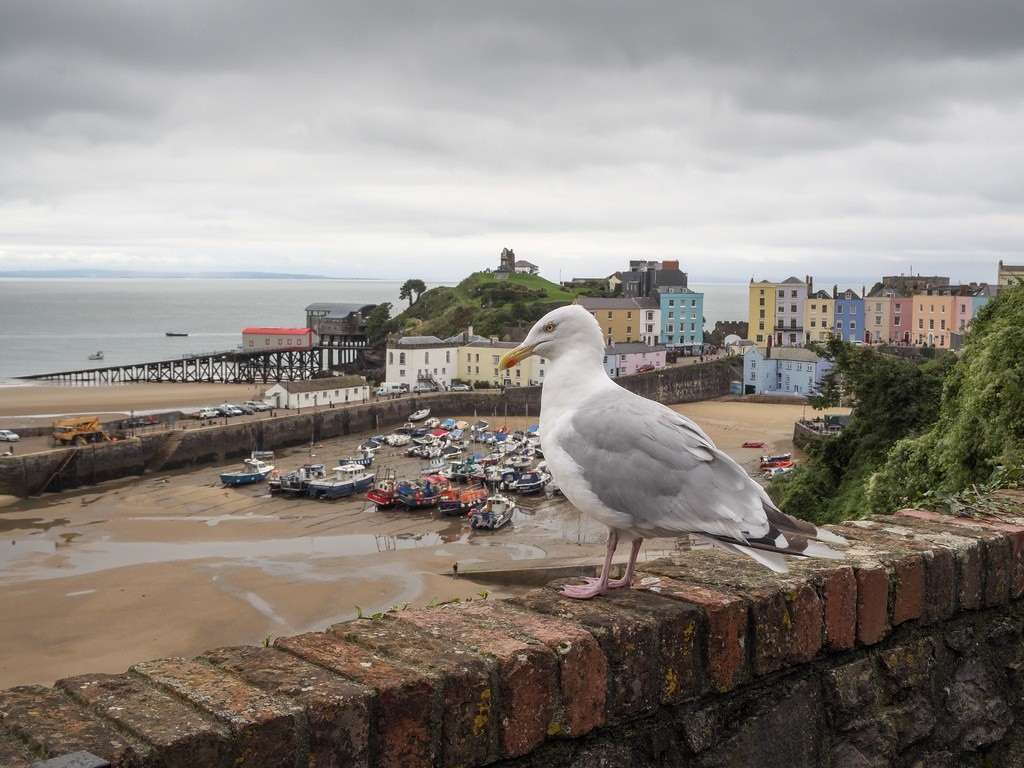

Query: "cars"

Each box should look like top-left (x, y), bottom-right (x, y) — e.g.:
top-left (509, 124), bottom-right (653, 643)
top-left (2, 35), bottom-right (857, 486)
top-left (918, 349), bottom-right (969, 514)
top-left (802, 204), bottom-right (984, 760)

top-left (236, 405), bottom-right (255, 415)
top-left (451, 383), bottom-right (471, 392)
top-left (414, 385), bottom-right (431, 393)
top-left (213, 408), bottom-right (232, 418)
top-left (0, 430), bottom-right (20, 441)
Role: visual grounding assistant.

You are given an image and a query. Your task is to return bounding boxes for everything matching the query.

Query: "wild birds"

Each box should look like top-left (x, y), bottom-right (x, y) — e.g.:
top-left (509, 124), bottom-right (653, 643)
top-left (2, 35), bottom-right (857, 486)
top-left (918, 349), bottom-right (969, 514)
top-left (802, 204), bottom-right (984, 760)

top-left (498, 304), bottom-right (850, 598)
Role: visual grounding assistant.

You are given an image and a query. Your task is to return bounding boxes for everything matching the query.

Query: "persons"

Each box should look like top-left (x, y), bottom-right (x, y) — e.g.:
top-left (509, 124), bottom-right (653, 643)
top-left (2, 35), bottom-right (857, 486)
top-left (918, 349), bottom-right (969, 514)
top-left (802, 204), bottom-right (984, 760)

top-left (453, 562), bottom-right (459, 580)
top-left (700, 354), bottom-right (720, 362)
top-left (10, 445), bottom-right (13, 454)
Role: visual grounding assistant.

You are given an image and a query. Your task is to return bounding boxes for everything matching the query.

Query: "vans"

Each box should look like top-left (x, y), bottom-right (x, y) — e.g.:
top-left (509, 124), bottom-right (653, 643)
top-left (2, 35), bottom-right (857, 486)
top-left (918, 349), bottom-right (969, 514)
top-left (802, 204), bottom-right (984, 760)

top-left (243, 401), bottom-right (267, 412)
top-left (219, 405), bottom-right (243, 416)
top-left (381, 381), bottom-right (408, 394)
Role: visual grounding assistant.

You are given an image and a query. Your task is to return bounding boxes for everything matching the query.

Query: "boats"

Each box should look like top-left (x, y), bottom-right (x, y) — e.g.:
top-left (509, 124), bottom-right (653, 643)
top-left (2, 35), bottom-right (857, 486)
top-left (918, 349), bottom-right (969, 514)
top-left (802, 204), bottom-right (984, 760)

top-left (270, 416), bottom-right (565, 530)
top-left (89, 352), bottom-right (104, 359)
top-left (742, 441), bottom-right (764, 448)
top-left (219, 451), bottom-right (275, 487)
top-left (407, 409), bottom-right (432, 421)
top-left (760, 453), bottom-right (795, 481)
top-left (165, 332), bottom-right (188, 337)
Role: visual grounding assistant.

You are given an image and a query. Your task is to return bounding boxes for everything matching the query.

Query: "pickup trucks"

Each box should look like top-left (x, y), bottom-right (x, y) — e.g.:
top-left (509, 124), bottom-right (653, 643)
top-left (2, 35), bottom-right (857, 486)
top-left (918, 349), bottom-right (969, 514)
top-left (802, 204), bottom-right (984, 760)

top-left (193, 408), bottom-right (220, 420)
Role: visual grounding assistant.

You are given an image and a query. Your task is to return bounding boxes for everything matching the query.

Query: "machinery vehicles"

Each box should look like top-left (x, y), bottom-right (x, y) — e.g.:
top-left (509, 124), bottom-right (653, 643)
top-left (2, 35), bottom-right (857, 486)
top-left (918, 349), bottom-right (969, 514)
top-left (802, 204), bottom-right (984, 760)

top-left (50, 416), bottom-right (115, 445)
